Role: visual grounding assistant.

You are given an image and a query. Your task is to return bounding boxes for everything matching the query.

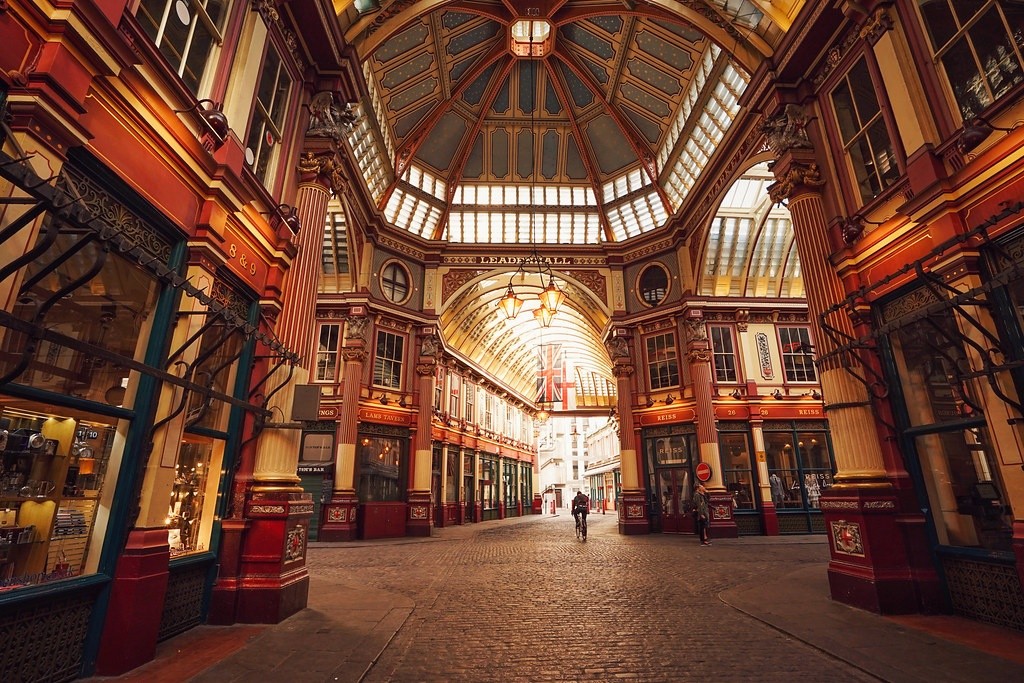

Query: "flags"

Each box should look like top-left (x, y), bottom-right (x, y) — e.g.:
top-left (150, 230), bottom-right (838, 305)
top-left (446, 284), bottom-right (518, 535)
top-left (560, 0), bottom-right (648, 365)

top-left (535, 343), bottom-right (577, 412)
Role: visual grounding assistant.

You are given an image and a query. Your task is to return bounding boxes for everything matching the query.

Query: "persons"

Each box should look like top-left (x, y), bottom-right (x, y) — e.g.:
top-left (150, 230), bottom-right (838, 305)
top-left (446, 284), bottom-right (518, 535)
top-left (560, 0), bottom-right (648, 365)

top-left (571, 490), bottom-right (589, 536)
top-left (770, 474), bottom-right (785, 508)
top-left (807, 476), bottom-right (821, 510)
top-left (661, 490), bottom-right (684, 515)
top-left (693, 482), bottom-right (713, 547)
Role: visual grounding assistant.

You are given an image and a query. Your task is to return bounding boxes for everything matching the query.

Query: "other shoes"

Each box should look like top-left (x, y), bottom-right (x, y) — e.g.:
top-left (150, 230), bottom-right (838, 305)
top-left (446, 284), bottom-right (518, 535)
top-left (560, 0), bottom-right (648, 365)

top-left (576, 522), bottom-right (580, 527)
top-left (705, 538), bottom-right (713, 542)
top-left (701, 542), bottom-right (712, 547)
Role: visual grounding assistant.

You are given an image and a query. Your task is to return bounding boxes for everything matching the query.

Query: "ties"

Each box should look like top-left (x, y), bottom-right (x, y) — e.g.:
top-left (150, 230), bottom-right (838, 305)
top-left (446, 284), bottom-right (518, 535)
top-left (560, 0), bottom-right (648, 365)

top-left (775, 478), bottom-right (778, 485)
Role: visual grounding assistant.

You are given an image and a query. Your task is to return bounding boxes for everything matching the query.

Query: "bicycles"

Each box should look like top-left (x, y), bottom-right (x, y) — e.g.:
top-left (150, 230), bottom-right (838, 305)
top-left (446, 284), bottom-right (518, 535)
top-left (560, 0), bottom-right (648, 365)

top-left (572, 511), bottom-right (588, 541)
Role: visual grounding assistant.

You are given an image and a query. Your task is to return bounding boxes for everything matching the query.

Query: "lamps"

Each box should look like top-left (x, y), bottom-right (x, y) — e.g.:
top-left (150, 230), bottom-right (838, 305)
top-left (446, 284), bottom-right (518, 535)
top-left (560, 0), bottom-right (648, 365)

top-left (376, 393), bottom-right (387, 406)
top-left (258, 203), bottom-right (301, 236)
top-left (646, 397), bottom-right (656, 408)
top-left (394, 396), bottom-right (407, 408)
top-left (666, 394), bottom-right (676, 405)
top-left (805, 389), bottom-right (821, 400)
top-left (496, 38), bottom-right (566, 327)
top-left (607, 405), bottom-right (620, 438)
top-left (771, 389), bottom-right (783, 400)
top-left (843, 214), bottom-right (882, 246)
top-left (957, 116), bottom-right (1015, 157)
top-left (729, 387), bottom-right (741, 400)
top-left (174, 98), bottom-right (229, 144)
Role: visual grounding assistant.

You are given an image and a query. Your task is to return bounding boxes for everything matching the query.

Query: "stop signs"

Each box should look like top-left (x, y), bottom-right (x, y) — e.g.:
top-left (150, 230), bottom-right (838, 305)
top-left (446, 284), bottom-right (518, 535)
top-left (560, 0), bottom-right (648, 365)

top-left (696, 462), bottom-right (711, 482)
